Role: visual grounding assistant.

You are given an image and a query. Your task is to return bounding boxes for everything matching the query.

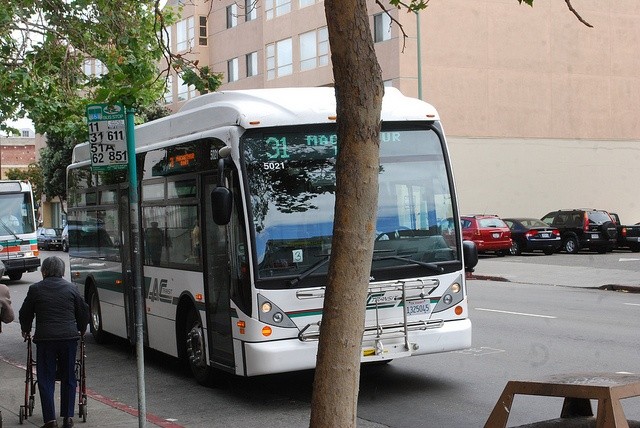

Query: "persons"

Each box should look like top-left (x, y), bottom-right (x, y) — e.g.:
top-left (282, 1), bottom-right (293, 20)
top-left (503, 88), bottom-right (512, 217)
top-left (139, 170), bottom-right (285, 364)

top-left (192, 219), bottom-right (200, 265)
top-left (0, 207), bottom-right (20, 235)
top-left (19, 256), bottom-right (87, 427)
top-left (146, 222), bottom-right (165, 268)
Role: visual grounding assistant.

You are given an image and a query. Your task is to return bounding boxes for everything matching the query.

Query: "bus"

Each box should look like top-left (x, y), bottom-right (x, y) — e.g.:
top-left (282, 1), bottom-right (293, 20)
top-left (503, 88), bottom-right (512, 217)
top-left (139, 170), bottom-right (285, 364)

top-left (65, 85), bottom-right (472, 386)
top-left (0, 179), bottom-right (41, 280)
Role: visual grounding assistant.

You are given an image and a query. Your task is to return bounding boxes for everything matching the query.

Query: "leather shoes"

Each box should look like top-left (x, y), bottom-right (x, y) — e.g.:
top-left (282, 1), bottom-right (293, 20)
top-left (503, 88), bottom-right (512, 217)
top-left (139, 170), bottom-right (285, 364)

top-left (62, 418), bottom-right (75, 428)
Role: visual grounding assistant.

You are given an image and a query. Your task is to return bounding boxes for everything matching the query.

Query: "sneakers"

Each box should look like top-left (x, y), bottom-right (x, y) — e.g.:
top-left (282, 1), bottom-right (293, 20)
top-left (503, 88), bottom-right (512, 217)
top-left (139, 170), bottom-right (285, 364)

top-left (41, 420), bottom-right (60, 428)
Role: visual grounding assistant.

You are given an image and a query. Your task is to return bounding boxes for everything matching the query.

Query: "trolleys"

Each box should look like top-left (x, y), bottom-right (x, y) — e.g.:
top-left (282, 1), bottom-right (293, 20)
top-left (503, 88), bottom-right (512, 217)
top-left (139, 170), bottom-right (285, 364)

top-left (18, 331), bottom-right (89, 424)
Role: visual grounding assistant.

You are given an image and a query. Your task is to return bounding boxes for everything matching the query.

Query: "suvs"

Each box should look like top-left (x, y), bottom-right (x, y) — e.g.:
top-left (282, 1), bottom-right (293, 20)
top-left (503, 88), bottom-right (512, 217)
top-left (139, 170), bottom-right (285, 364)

top-left (438, 214), bottom-right (513, 257)
top-left (534, 208), bottom-right (619, 254)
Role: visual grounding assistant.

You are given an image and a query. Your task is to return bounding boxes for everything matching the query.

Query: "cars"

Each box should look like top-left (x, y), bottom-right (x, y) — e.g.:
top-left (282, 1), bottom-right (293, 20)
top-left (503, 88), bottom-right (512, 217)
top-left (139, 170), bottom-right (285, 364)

top-left (36, 226), bottom-right (64, 251)
top-left (502, 217), bottom-right (562, 256)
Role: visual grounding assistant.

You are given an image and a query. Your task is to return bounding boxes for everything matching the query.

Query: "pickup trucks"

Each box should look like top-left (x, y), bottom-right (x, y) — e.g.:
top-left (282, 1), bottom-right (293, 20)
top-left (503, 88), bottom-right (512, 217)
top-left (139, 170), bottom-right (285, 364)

top-left (609, 212), bottom-right (640, 253)
